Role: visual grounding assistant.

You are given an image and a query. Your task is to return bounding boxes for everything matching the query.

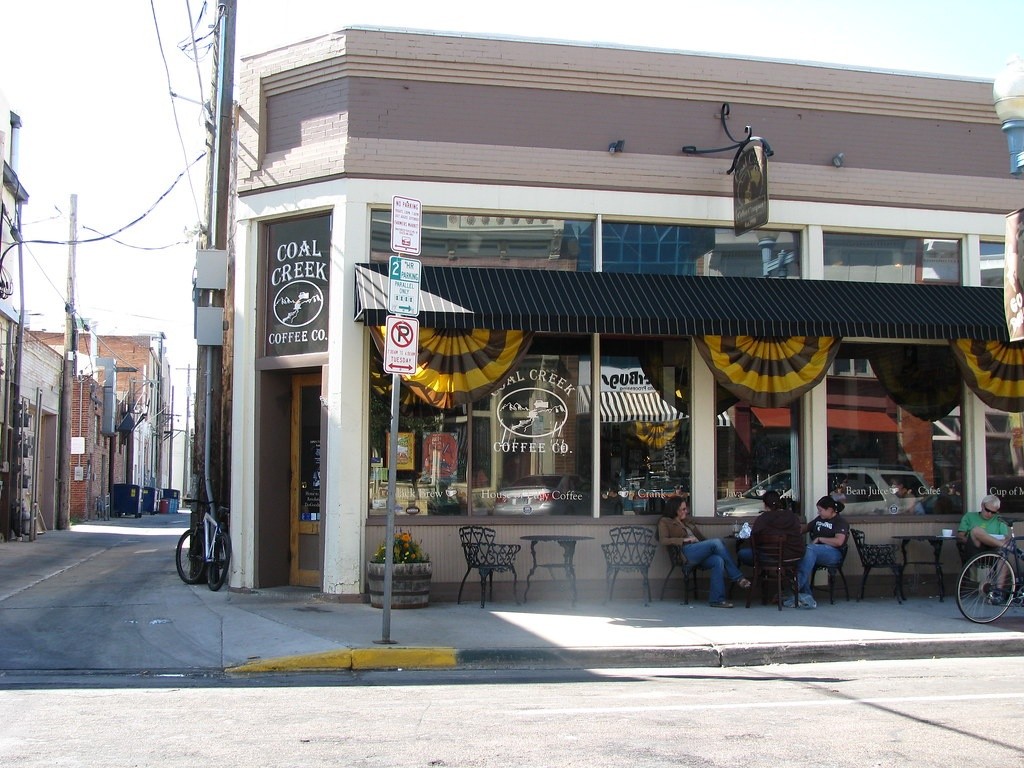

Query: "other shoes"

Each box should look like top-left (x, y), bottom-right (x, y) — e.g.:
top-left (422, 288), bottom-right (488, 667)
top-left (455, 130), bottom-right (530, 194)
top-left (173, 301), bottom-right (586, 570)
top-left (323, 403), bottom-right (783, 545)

top-left (992, 597), bottom-right (1003, 604)
top-left (1017, 548), bottom-right (1024, 560)
top-left (711, 601), bottom-right (734, 607)
top-left (798, 593), bottom-right (816, 608)
top-left (784, 596), bottom-right (795, 607)
top-left (736, 578), bottom-right (751, 591)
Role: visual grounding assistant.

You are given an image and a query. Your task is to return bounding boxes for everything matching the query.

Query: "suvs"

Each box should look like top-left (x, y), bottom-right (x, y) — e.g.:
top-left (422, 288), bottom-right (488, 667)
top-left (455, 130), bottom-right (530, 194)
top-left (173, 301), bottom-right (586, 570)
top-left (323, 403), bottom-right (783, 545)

top-left (715, 463), bottom-right (934, 515)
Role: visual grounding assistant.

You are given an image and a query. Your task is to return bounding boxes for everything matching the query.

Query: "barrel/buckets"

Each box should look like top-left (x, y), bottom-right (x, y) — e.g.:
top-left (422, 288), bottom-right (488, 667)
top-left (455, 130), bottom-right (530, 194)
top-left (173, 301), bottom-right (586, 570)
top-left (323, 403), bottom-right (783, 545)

top-left (160, 499), bottom-right (177, 514)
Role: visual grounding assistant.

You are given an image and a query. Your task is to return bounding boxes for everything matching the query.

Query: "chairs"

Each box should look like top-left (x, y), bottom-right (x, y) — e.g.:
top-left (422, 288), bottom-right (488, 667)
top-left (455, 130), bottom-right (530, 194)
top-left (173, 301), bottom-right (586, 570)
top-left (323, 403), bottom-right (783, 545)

top-left (601, 526), bottom-right (654, 604)
top-left (457, 526), bottom-right (521, 608)
top-left (810, 532), bottom-right (850, 604)
top-left (655, 527), bottom-right (714, 604)
top-left (958, 534), bottom-right (999, 597)
top-left (743, 534), bottom-right (799, 612)
top-left (850, 528), bottom-right (907, 604)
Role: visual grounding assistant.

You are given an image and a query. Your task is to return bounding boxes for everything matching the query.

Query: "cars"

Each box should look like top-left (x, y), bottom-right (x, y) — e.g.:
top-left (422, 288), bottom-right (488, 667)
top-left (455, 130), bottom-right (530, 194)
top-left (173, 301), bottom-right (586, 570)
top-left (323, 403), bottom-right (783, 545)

top-left (493, 473), bottom-right (593, 516)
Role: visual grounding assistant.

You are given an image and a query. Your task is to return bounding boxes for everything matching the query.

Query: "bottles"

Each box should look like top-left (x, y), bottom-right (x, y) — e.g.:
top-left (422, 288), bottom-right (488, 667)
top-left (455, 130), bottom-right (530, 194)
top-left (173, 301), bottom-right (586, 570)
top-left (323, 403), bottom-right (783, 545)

top-left (733, 520), bottom-right (739, 538)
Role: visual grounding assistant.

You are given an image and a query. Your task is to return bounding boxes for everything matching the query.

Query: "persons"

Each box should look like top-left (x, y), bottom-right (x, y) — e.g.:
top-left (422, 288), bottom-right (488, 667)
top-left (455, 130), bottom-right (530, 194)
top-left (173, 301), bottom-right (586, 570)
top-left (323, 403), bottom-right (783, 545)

top-left (888, 476), bottom-right (926, 516)
top-left (737, 490), bottom-right (806, 603)
top-left (957, 495), bottom-right (1024, 604)
top-left (541, 463), bottom-right (623, 514)
top-left (830, 473), bottom-right (848, 505)
top-left (659, 496), bottom-right (751, 607)
top-left (782, 496), bottom-right (850, 609)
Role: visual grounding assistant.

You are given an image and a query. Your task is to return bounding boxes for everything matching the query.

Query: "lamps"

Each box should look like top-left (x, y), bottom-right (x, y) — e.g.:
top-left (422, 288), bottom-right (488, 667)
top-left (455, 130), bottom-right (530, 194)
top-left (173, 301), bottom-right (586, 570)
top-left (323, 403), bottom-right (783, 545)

top-left (607, 139), bottom-right (626, 153)
top-left (830, 152), bottom-right (845, 168)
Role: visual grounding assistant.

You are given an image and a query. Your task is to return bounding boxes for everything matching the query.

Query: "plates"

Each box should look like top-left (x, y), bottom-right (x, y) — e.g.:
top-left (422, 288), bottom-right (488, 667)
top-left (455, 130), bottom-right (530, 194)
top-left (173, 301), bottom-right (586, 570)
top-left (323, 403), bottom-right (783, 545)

top-left (937, 535), bottom-right (957, 538)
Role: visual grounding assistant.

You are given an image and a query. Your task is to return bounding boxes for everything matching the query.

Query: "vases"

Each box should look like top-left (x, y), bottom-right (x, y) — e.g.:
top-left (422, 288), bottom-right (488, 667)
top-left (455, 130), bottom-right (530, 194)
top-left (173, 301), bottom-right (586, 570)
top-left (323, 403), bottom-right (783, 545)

top-left (368, 562), bottom-right (433, 607)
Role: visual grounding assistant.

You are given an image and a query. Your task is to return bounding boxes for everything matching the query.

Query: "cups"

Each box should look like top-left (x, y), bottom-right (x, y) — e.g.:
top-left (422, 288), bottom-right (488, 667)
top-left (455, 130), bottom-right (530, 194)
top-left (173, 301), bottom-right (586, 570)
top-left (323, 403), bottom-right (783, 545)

top-left (942, 529), bottom-right (952, 536)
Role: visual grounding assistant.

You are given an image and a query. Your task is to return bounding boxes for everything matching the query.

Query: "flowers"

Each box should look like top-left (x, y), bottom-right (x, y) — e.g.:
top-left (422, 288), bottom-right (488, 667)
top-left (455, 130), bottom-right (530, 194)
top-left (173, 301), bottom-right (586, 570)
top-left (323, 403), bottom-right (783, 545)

top-left (369, 529), bottom-right (431, 563)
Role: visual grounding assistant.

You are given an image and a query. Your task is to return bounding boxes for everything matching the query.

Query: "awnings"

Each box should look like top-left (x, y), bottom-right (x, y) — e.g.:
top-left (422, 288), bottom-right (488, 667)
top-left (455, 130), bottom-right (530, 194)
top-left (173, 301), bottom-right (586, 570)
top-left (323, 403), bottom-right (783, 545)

top-left (928, 413), bottom-right (1015, 439)
top-left (353, 261), bottom-right (1012, 341)
top-left (576, 383), bottom-right (732, 430)
top-left (748, 407), bottom-right (905, 433)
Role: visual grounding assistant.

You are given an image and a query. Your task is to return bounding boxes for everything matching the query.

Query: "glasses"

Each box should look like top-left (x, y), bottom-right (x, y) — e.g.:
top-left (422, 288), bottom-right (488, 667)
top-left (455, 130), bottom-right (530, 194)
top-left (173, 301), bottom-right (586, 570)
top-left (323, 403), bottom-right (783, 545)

top-left (984, 506), bottom-right (996, 514)
top-left (679, 508), bottom-right (686, 511)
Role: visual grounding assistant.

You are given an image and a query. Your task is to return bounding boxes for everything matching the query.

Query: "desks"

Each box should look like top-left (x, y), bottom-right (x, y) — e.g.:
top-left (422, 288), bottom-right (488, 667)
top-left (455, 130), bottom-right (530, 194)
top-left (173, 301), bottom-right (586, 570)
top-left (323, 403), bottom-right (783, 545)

top-left (722, 533), bottom-right (754, 604)
top-left (889, 535), bottom-right (961, 602)
top-left (519, 536), bottom-right (595, 607)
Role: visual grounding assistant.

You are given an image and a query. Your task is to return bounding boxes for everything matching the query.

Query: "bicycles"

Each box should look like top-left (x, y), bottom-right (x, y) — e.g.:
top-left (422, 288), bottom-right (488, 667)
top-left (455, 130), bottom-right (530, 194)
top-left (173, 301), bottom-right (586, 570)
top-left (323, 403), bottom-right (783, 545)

top-left (175, 499), bottom-right (235, 593)
top-left (955, 516), bottom-right (1024, 624)
